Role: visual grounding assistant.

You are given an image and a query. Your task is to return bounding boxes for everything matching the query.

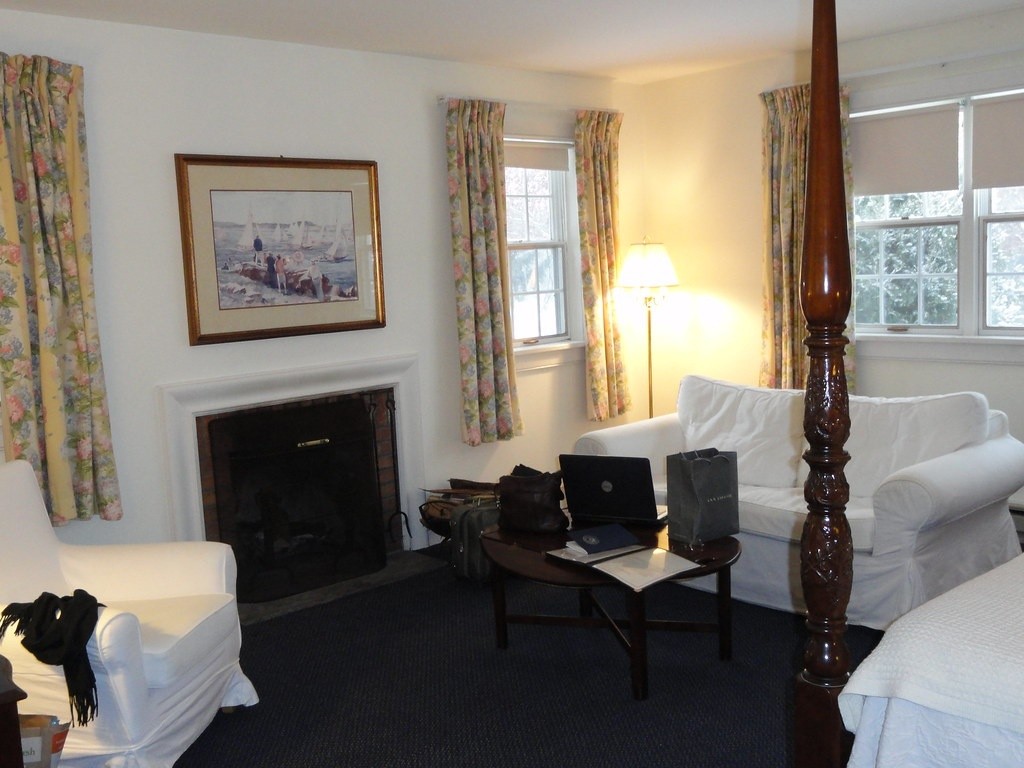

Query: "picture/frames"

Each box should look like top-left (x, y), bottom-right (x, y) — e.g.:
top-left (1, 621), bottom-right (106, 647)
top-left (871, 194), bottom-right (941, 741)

top-left (174, 153), bottom-right (386, 350)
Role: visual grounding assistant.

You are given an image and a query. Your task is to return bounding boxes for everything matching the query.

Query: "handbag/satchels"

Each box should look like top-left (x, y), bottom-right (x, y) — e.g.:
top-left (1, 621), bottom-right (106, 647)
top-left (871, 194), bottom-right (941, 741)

top-left (452, 491), bottom-right (499, 578)
top-left (493, 464), bottom-right (569, 535)
top-left (666, 447), bottom-right (739, 548)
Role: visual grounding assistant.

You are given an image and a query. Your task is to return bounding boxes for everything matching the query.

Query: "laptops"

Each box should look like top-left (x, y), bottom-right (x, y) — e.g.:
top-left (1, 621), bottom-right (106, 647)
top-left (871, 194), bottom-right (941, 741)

top-left (560, 455), bottom-right (668, 524)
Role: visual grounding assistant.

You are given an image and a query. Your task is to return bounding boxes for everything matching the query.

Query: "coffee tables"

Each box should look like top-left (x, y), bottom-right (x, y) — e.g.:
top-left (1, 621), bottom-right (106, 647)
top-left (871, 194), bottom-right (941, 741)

top-left (479, 509), bottom-right (743, 701)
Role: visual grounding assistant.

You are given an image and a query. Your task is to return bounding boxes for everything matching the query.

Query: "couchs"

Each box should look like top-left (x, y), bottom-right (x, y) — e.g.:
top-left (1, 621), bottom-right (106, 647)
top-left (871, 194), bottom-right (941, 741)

top-left (574, 374), bottom-right (1024, 633)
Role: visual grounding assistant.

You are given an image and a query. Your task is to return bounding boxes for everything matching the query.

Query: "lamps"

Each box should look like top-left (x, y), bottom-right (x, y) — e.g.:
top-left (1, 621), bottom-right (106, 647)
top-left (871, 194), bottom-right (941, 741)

top-left (615, 241), bottom-right (679, 419)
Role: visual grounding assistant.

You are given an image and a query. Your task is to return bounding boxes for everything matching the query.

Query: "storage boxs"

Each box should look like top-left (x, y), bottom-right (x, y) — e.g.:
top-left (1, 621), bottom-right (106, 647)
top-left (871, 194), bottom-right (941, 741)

top-left (16, 714), bottom-right (73, 768)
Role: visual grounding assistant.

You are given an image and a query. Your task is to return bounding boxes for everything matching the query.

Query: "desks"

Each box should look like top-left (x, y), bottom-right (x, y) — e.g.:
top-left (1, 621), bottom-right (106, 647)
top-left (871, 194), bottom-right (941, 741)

top-left (837, 554), bottom-right (1024, 767)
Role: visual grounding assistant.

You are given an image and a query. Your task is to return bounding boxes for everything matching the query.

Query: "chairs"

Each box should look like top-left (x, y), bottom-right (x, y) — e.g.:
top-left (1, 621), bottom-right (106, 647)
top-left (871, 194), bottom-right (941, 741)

top-left (0, 459), bottom-right (259, 768)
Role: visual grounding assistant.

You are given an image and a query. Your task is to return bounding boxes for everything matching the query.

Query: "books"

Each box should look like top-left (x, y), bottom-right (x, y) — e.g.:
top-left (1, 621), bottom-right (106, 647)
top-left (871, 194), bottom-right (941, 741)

top-left (566, 523), bottom-right (639, 554)
